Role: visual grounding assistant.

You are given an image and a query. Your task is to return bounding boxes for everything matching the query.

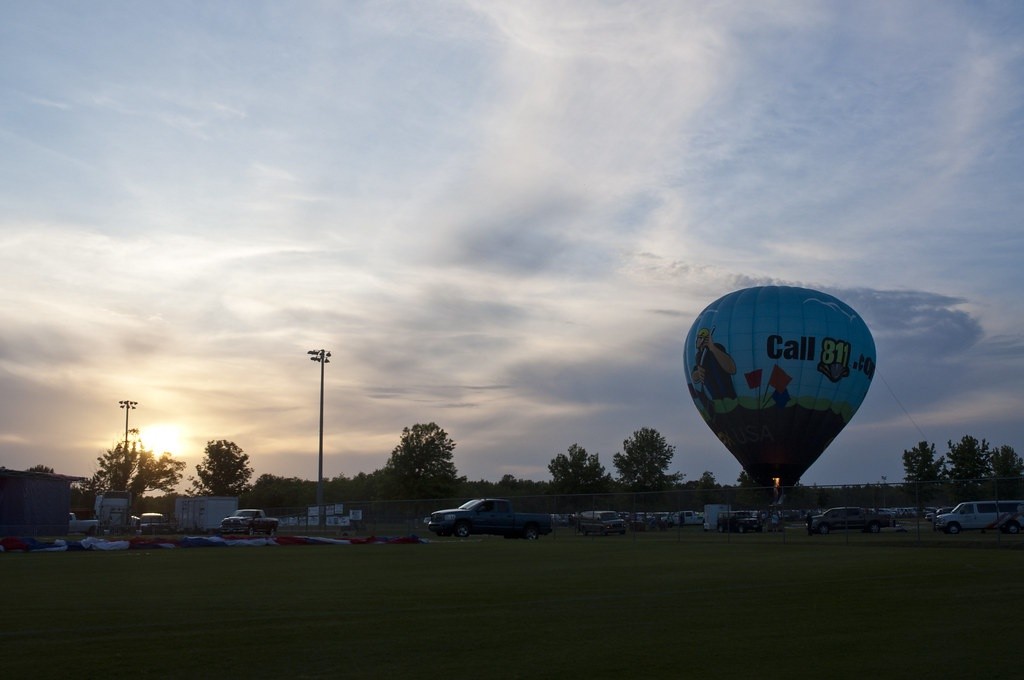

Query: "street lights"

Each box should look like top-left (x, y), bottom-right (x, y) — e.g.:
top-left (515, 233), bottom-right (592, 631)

top-left (119, 400), bottom-right (138, 462)
top-left (307, 349), bottom-right (331, 528)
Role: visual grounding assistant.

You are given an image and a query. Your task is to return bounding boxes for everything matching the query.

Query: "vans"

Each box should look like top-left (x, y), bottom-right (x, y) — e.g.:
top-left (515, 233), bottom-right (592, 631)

top-left (579, 510), bottom-right (628, 536)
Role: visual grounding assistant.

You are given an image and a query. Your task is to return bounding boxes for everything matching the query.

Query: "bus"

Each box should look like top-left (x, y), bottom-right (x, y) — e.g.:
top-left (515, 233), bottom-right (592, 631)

top-left (140, 513), bottom-right (165, 534)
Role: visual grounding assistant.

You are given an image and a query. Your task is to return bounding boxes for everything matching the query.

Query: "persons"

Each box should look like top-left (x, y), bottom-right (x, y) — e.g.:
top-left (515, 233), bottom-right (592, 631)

top-left (805, 513), bottom-right (813, 536)
top-left (938, 510), bottom-right (943, 515)
top-left (678, 512), bottom-right (685, 528)
top-left (568, 512), bottom-right (576, 527)
top-left (772, 512), bottom-right (779, 534)
top-left (932, 510), bottom-right (937, 531)
top-left (667, 512), bottom-right (673, 528)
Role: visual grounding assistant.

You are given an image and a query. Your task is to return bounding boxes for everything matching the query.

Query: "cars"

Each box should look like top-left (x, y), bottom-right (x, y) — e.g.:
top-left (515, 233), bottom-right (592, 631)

top-left (131, 515), bottom-right (140, 526)
top-left (871, 506), bottom-right (938, 519)
top-left (551, 514), bottom-right (577, 528)
top-left (617, 510), bottom-right (784, 536)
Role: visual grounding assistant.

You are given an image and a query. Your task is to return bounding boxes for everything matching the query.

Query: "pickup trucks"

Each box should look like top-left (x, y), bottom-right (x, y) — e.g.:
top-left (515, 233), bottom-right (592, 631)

top-left (219, 509), bottom-right (279, 536)
top-left (427, 498), bottom-right (553, 541)
top-left (68, 513), bottom-right (100, 536)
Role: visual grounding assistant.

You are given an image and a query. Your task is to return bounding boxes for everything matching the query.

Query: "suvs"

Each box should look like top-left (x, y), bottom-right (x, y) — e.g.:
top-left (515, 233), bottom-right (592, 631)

top-left (924, 506), bottom-right (955, 522)
top-left (936, 501), bottom-right (1022, 535)
top-left (806, 508), bottom-right (882, 535)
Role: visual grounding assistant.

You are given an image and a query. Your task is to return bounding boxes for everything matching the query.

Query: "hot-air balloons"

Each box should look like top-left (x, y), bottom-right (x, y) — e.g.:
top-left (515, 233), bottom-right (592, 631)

top-left (683, 285), bottom-right (878, 508)
